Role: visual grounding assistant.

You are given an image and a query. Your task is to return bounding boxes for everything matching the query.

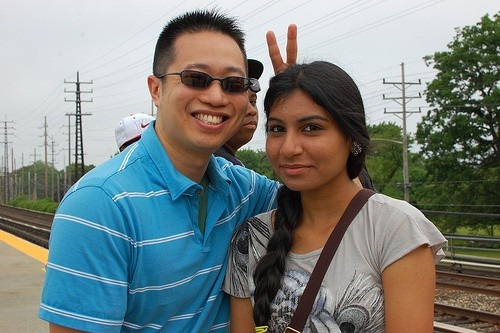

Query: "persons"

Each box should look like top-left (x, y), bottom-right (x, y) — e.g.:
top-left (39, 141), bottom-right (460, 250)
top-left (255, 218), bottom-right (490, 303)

top-left (38, 11), bottom-right (298, 333)
top-left (213, 58), bottom-right (264, 167)
top-left (223, 61), bottom-right (449, 333)
top-left (114, 112), bottom-right (156, 152)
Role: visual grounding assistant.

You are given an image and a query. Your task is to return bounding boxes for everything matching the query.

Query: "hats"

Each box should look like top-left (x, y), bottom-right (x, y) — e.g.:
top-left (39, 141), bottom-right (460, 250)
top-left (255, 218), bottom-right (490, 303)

top-left (114, 113), bottom-right (157, 151)
top-left (246, 58), bottom-right (263, 81)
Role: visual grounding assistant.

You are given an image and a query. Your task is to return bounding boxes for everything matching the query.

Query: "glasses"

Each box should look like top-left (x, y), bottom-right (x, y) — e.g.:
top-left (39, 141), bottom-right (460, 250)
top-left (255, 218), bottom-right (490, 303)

top-left (248, 77), bottom-right (261, 92)
top-left (155, 69), bottom-right (253, 94)
